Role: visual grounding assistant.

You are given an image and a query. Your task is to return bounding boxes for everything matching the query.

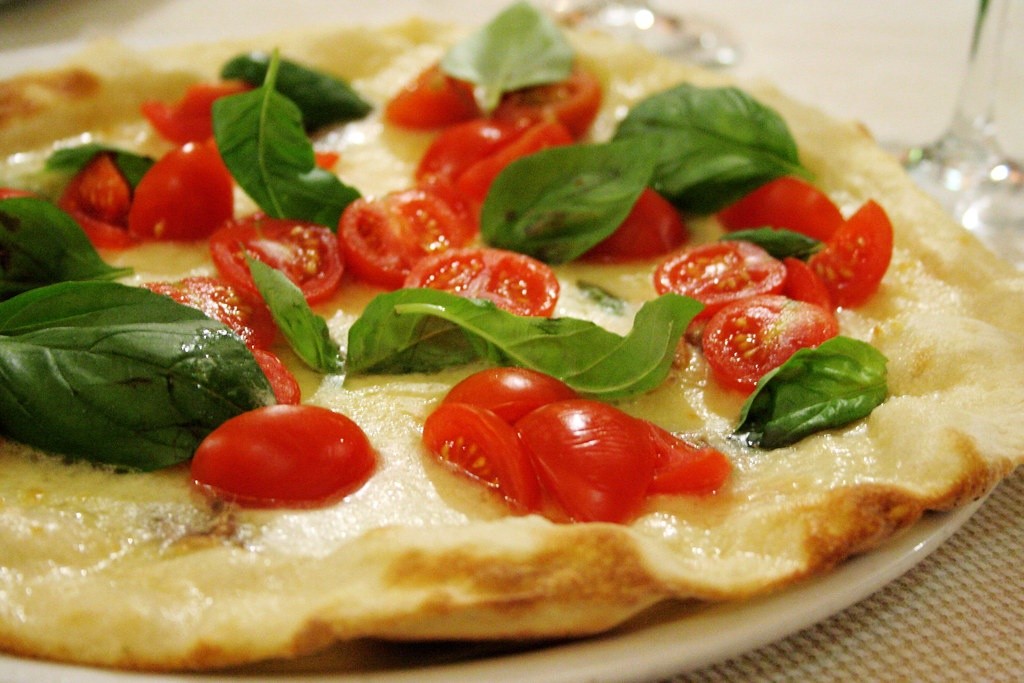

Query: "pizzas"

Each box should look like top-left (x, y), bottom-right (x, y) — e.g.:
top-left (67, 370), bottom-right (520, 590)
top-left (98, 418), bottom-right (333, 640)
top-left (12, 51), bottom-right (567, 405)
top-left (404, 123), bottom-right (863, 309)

top-left (0, 0), bottom-right (1024, 672)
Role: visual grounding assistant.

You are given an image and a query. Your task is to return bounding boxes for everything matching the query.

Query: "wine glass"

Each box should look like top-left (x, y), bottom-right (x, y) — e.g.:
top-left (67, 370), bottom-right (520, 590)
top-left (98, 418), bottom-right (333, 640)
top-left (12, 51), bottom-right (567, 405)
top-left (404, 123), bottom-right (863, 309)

top-left (893, 0), bottom-right (1024, 272)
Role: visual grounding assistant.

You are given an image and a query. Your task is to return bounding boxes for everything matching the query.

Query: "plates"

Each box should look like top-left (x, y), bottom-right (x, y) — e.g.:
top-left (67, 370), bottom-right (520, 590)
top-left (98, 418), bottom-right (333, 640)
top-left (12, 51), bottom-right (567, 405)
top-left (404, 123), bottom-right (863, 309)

top-left (0, 45), bottom-right (1000, 683)
